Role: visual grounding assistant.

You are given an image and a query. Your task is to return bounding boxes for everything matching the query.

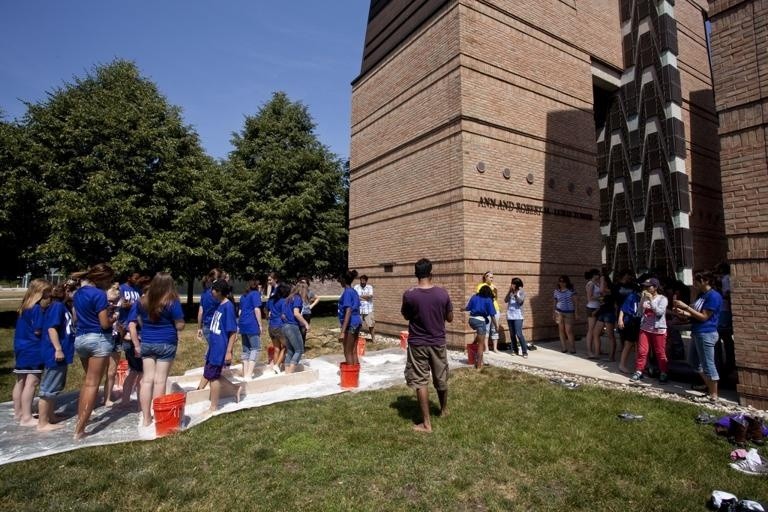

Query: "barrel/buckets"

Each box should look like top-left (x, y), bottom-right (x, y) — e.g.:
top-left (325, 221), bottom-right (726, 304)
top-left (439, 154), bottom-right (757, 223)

top-left (339, 361), bottom-right (360, 389)
top-left (467, 343), bottom-right (480, 365)
top-left (153, 392), bottom-right (188, 438)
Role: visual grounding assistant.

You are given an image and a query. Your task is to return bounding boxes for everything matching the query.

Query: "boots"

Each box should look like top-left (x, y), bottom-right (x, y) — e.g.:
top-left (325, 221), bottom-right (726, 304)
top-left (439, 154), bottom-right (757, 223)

top-left (719, 418), bottom-right (749, 446)
top-left (744, 416), bottom-right (764, 445)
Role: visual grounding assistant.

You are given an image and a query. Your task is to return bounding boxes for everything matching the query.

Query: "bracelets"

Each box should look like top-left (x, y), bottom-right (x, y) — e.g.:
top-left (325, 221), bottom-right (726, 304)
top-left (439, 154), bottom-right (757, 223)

top-left (683, 304), bottom-right (693, 311)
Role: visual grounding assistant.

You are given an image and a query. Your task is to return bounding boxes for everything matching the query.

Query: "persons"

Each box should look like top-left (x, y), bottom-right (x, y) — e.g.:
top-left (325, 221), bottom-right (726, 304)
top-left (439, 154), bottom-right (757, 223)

top-left (267, 272), bottom-right (280, 320)
top-left (12, 278), bottom-right (52, 428)
top-left (665, 279), bottom-right (691, 350)
top-left (503, 277), bottom-right (529, 359)
top-left (262, 284), bottom-right (290, 374)
top-left (203, 279), bottom-right (242, 413)
top-left (291, 278), bottom-right (320, 343)
top-left (587, 275), bottom-right (616, 362)
top-left (672, 268), bottom-right (724, 403)
top-left (118, 270), bottom-right (145, 323)
top-left (281, 281), bottom-right (310, 374)
top-left (221, 270), bottom-right (231, 283)
top-left (72, 264), bottom-right (120, 440)
top-left (353, 275), bottom-right (376, 345)
top-left (102, 277), bottom-right (122, 407)
top-left (197, 268), bottom-right (240, 391)
top-left (121, 284), bottom-right (153, 413)
top-left (711, 262), bottom-right (737, 376)
top-left (38, 278), bottom-right (81, 432)
top-left (610, 270), bottom-right (641, 354)
top-left (135, 276), bottom-right (153, 295)
top-left (552, 274), bottom-right (579, 354)
top-left (617, 273), bottom-right (653, 374)
top-left (631, 277), bottom-right (670, 385)
top-left (237, 278), bottom-right (265, 382)
top-left (476, 271), bottom-right (501, 355)
top-left (584, 268), bottom-right (604, 357)
top-left (460, 285), bottom-right (498, 372)
top-left (135, 271), bottom-right (185, 426)
top-left (400, 259), bottom-right (454, 434)
top-left (335, 269), bottom-right (362, 366)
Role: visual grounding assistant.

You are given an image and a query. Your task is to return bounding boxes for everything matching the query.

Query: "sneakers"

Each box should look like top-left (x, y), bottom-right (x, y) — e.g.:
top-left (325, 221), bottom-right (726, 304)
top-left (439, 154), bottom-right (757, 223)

top-left (659, 373), bottom-right (669, 385)
top-left (691, 394), bottom-right (719, 405)
top-left (731, 460), bottom-right (767, 475)
top-left (632, 371), bottom-right (644, 381)
top-left (692, 384), bottom-right (709, 393)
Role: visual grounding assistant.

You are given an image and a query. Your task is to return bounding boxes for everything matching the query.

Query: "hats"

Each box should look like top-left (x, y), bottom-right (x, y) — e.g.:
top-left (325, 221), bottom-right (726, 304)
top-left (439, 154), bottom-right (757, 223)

top-left (641, 279), bottom-right (660, 287)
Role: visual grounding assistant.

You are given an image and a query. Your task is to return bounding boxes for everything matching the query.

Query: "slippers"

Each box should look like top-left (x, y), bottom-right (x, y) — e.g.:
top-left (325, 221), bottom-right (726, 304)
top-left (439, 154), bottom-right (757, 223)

top-left (618, 413), bottom-right (643, 421)
top-left (696, 415), bottom-right (717, 424)
